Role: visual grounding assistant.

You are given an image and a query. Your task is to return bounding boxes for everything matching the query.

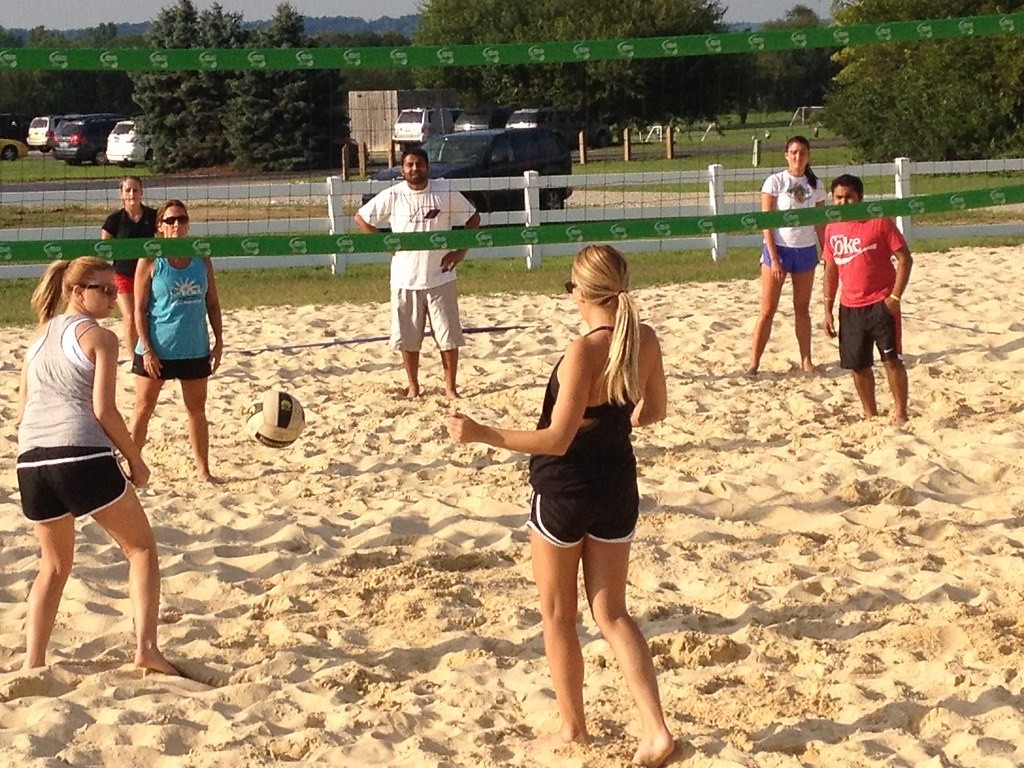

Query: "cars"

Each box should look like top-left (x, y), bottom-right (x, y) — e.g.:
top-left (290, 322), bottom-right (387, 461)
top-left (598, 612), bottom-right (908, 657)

top-left (0, 138), bottom-right (27, 161)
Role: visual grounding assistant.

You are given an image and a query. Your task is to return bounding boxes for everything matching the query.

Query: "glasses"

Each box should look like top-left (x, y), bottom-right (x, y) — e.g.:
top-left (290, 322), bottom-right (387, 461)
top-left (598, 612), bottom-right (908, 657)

top-left (71, 281), bottom-right (118, 297)
top-left (564, 280), bottom-right (578, 294)
top-left (161, 215), bottom-right (190, 225)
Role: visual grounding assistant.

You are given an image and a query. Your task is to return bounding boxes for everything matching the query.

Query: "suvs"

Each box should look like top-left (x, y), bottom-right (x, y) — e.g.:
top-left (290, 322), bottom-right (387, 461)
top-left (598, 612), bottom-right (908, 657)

top-left (390, 107), bottom-right (614, 152)
top-left (27, 112), bottom-right (154, 168)
top-left (361, 125), bottom-right (575, 232)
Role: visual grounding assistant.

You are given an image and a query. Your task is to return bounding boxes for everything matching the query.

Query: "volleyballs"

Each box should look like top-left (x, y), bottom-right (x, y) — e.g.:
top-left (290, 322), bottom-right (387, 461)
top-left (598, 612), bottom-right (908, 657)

top-left (245, 391), bottom-right (304, 449)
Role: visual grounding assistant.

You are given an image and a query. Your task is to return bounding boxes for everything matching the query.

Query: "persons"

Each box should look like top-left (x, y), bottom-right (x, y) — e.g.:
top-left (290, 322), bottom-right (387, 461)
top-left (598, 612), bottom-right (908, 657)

top-left (747, 136), bottom-right (914, 423)
top-left (445, 244), bottom-right (674, 768)
top-left (355, 146), bottom-right (481, 401)
top-left (16, 177), bottom-right (222, 677)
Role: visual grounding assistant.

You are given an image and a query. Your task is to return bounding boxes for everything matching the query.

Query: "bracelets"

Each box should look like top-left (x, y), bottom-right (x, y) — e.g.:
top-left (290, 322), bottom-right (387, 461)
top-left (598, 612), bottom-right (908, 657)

top-left (140, 347), bottom-right (153, 356)
top-left (889, 294), bottom-right (901, 303)
